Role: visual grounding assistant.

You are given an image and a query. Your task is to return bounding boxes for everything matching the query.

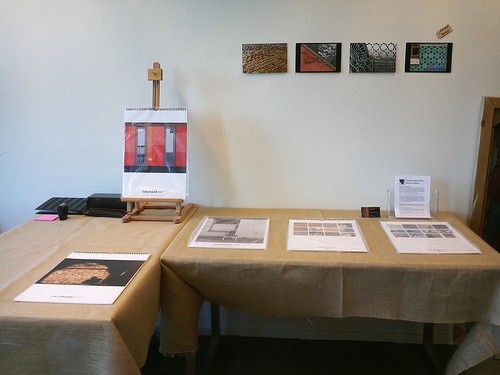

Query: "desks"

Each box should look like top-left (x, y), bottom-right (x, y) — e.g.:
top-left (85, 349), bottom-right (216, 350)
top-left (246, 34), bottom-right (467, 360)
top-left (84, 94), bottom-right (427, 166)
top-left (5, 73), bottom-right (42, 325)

top-left (0, 204), bottom-right (198, 374)
top-left (161, 207), bottom-right (499, 374)
